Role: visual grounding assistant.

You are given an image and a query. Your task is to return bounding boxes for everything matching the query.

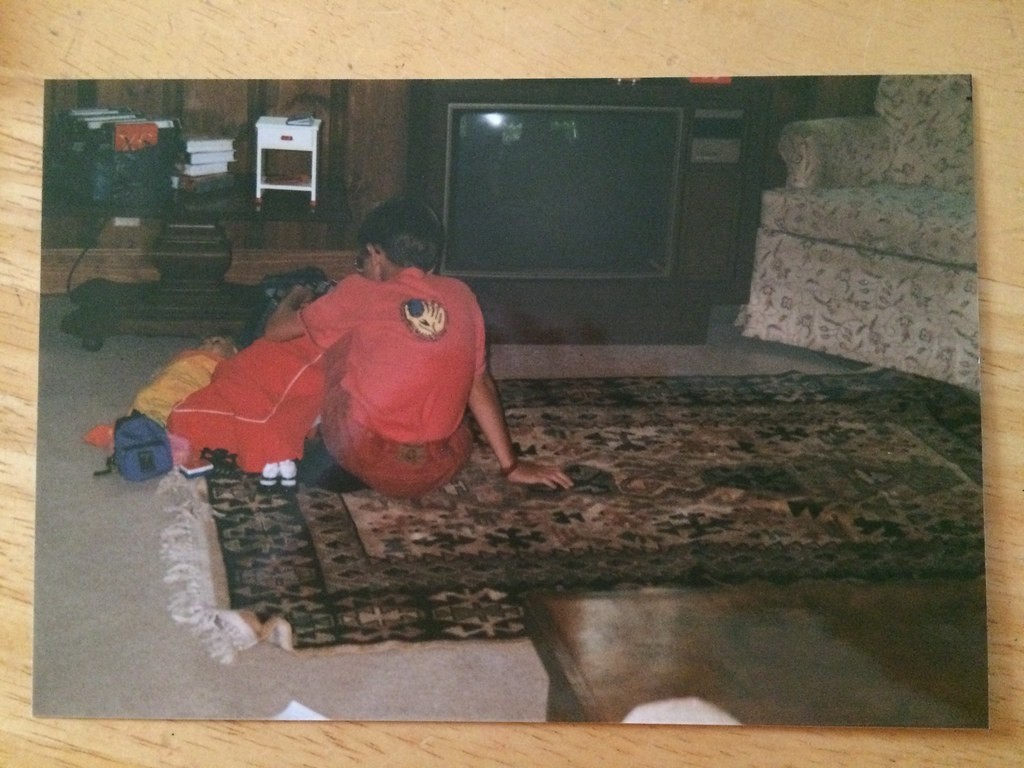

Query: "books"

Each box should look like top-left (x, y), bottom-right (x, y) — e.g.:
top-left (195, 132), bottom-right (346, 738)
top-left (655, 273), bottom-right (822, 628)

top-left (54, 109), bottom-right (248, 224)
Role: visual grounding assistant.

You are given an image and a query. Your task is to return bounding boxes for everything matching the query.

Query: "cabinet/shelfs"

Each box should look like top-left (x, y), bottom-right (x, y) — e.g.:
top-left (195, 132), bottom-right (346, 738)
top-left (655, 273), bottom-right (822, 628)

top-left (254, 115), bottom-right (323, 214)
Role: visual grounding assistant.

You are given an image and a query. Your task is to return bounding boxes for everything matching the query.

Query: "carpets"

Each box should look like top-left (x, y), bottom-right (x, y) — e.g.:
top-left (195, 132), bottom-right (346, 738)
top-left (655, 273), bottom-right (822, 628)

top-left (160, 366), bottom-right (985, 666)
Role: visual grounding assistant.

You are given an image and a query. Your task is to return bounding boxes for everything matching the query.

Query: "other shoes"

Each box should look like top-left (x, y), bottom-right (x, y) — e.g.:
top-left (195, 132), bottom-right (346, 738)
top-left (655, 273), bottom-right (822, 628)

top-left (257, 458), bottom-right (299, 495)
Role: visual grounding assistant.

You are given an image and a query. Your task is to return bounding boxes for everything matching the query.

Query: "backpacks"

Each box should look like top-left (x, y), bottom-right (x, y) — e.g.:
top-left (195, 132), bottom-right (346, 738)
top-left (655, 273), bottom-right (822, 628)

top-left (95, 409), bottom-right (173, 482)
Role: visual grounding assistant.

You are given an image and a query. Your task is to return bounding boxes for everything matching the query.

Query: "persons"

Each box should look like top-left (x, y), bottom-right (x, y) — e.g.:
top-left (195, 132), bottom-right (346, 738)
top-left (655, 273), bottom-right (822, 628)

top-left (267, 194), bottom-right (574, 499)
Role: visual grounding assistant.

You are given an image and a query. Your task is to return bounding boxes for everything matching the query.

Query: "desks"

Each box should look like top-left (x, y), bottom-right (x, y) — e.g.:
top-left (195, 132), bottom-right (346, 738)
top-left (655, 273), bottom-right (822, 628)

top-left (516, 588), bottom-right (989, 730)
top-left (53, 170), bottom-right (351, 356)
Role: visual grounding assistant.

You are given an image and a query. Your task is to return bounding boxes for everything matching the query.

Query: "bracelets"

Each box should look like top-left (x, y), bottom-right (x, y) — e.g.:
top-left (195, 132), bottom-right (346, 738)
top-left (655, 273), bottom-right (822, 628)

top-left (503, 458), bottom-right (519, 476)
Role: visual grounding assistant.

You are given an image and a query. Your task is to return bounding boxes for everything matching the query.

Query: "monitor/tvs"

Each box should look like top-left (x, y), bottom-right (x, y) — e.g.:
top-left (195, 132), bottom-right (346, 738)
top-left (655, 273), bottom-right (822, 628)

top-left (441, 103), bottom-right (686, 279)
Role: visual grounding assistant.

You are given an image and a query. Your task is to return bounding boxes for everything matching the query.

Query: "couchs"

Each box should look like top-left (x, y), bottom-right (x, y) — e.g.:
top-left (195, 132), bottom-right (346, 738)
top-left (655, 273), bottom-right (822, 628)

top-left (733, 76), bottom-right (982, 395)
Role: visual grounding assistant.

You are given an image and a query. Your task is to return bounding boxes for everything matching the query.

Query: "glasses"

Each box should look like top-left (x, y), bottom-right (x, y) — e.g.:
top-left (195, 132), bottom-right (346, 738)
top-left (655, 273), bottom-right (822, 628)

top-left (353, 250), bottom-right (380, 272)
